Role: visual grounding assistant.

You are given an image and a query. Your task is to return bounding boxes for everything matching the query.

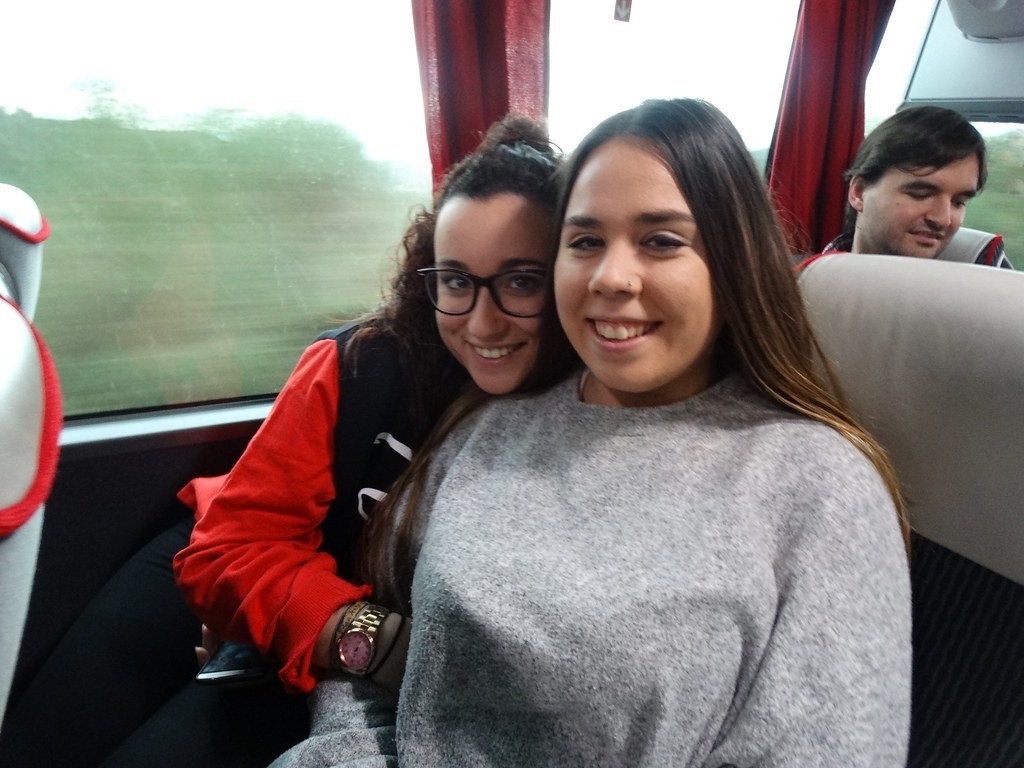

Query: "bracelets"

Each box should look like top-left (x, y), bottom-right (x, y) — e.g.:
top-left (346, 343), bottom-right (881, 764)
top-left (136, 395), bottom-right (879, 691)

top-left (367, 615), bottom-right (406, 673)
top-left (329, 600), bottom-right (368, 663)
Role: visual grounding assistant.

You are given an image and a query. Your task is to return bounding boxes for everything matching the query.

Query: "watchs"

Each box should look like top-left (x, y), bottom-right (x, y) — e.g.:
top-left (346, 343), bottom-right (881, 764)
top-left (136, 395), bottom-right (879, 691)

top-left (337, 604), bottom-right (390, 677)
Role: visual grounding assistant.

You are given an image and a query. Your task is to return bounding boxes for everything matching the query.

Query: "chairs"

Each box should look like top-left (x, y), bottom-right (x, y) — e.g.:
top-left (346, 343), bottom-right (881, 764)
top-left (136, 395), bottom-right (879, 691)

top-left (787, 251), bottom-right (1024, 768)
top-left (0, 181), bottom-right (64, 727)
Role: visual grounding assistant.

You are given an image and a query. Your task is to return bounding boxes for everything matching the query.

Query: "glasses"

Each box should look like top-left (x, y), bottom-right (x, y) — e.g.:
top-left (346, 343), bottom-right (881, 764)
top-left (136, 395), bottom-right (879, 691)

top-left (417, 268), bottom-right (553, 318)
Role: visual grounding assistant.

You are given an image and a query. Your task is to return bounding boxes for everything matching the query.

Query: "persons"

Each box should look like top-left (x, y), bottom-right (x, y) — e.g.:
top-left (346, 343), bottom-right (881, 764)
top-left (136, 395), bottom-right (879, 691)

top-left (822, 105), bottom-right (1015, 271)
top-left (0, 98), bottom-right (913, 768)
